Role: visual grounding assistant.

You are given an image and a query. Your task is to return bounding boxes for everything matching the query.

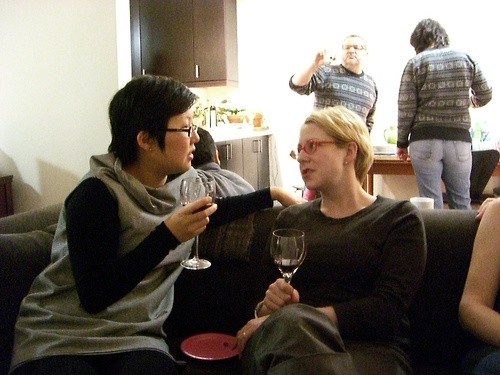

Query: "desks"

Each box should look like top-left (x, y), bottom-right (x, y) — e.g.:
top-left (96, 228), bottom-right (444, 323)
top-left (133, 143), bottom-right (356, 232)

top-left (367, 155), bottom-right (500, 196)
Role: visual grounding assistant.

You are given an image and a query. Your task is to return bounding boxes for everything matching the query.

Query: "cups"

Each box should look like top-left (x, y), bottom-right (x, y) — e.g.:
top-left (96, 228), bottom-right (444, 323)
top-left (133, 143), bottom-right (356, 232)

top-left (410, 197), bottom-right (434, 209)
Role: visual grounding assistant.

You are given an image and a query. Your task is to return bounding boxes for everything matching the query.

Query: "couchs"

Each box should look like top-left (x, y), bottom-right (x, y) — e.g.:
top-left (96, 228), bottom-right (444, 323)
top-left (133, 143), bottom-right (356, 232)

top-left (0, 201), bottom-right (500, 375)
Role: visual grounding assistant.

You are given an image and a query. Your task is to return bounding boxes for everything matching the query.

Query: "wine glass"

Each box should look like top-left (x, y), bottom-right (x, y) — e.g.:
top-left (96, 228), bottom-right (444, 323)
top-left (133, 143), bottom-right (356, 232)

top-left (179, 176), bottom-right (215, 270)
top-left (270, 228), bottom-right (307, 284)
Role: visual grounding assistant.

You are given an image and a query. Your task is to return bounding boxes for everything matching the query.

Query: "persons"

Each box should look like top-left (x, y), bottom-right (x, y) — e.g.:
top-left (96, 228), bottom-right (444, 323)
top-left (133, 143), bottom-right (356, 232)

top-left (289, 35), bottom-right (378, 200)
top-left (191, 128), bottom-right (254, 197)
top-left (10, 76), bottom-right (306, 374)
top-left (232, 105), bottom-right (427, 375)
top-left (457, 196), bottom-right (500, 375)
top-left (397, 18), bottom-right (492, 210)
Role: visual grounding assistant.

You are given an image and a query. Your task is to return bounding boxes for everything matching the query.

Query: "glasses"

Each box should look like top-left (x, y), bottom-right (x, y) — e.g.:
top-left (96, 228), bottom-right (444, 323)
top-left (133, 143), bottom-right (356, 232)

top-left (166, 126), bottom-right (199, 137)
top-left (298, 139), bottom-right (339, 154)
top-left (342, 45), bottom-right (365, 51)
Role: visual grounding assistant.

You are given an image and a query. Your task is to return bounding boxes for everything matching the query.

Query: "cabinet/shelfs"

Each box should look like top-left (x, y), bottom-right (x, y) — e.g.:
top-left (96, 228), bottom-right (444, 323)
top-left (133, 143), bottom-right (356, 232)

top-left (129, 1), bottom-right (238, 87)
top-left (202, 127), bottom-right (272, 192)
